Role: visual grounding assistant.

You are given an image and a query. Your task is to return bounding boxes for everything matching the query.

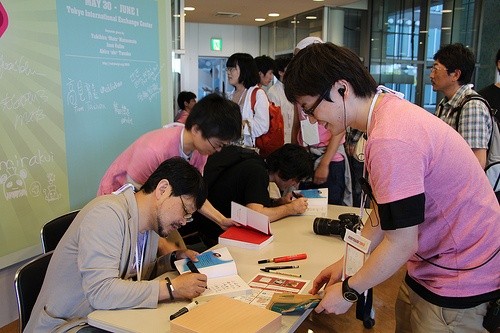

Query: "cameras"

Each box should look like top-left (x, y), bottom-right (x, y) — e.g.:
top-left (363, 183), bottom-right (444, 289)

top-left (313, 213), bottom-right (361, 239)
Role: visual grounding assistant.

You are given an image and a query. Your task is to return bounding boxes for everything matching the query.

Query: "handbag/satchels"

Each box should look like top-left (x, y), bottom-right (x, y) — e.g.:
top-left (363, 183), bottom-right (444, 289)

top-left (251, 89), bottom-right (285, 155)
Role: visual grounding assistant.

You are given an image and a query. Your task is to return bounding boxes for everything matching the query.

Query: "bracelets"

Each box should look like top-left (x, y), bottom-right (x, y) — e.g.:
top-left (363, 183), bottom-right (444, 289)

top-left (165, 277), bottom-right (175, 301)
top-left (170, 251), bottom-right (178, 270)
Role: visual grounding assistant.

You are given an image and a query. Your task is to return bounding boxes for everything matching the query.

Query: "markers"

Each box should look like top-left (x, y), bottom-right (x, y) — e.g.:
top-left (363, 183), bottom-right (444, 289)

top-left (258, 258), bottom-right (275, 264)
top-left (274, 254), bottom-right (307, 263)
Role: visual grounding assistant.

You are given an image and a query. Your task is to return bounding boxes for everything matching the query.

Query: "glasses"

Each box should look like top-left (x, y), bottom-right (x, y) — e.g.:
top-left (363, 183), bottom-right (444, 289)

top-left (301, 86), bottom-right (333, 119)
top-left (178, 195), bottom-right (193, 225)
top-left (431, 66), bottom-right (452, 72)
top-left (205, 136), bottom-right (224, 155)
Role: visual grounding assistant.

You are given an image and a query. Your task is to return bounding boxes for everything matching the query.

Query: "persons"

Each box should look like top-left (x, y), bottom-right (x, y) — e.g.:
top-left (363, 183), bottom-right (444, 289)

top-left (101, 93), bottom-right (242, 259)
top-left (429, 41), bottom-right (500, 205)
top-left (226, 37), bottom-right (371, 209)
top-left (284, 41), bottom-right (500, 333)
top-left (32, 155), bottom-right (207, 333)
top-left (172, 67), bottom-right (224, 125)
top-left (192, 142), bottom-right (315, 250)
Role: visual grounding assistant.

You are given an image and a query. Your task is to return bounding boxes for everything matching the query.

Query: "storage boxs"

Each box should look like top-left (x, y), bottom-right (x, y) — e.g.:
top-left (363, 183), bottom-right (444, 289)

top-left (171, 295), bottom-right (282, 333)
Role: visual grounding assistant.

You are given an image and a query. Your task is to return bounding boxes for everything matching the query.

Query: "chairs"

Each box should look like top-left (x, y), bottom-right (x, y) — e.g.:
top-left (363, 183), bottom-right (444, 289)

top-left (39, 209), bottom-right (82, 253)
top-left (13, 250), bottom-right (54, 333)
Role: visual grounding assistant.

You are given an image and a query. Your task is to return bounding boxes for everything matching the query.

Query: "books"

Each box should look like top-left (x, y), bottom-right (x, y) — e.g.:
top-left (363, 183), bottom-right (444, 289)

top-left (288, 187), bottom-right (328, 218)
top-left (264, 292), bottom-right (325, 315)
top-left (218, 201), bottom-right (273, 251)
top-left (174, 243), bottom-right (253, 304)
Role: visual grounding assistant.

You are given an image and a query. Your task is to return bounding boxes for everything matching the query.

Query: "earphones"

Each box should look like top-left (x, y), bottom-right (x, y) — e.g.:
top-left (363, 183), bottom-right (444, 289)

top-left (338, 88), bottom-right (345, 99)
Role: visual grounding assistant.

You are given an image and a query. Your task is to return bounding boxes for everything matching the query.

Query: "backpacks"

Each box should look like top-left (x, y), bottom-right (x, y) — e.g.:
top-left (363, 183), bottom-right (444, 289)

top-left (454, 95), bottom-right (500, 193)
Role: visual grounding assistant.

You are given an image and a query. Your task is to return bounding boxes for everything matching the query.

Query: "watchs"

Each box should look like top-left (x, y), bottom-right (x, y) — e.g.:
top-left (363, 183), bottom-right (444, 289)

top-left (341, 275), bottom-right (366, 303)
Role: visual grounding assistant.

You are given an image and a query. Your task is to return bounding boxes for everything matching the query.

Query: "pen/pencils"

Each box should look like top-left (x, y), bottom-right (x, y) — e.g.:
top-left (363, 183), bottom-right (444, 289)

top-left (187, 261), bottom-right (207, 289)
top-left (265, 266), bottom-right (299, 270)
top-left (260, 269), bottom-right (301, 278)
top-left (292, 191), bottom-right (299, 199)
top-left (170, 301), bottom-right (198, 320)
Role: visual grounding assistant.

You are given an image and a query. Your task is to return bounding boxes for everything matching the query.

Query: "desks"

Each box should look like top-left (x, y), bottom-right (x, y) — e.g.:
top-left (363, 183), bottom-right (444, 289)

top-left (88, 204), bottom-right (376, 333)
